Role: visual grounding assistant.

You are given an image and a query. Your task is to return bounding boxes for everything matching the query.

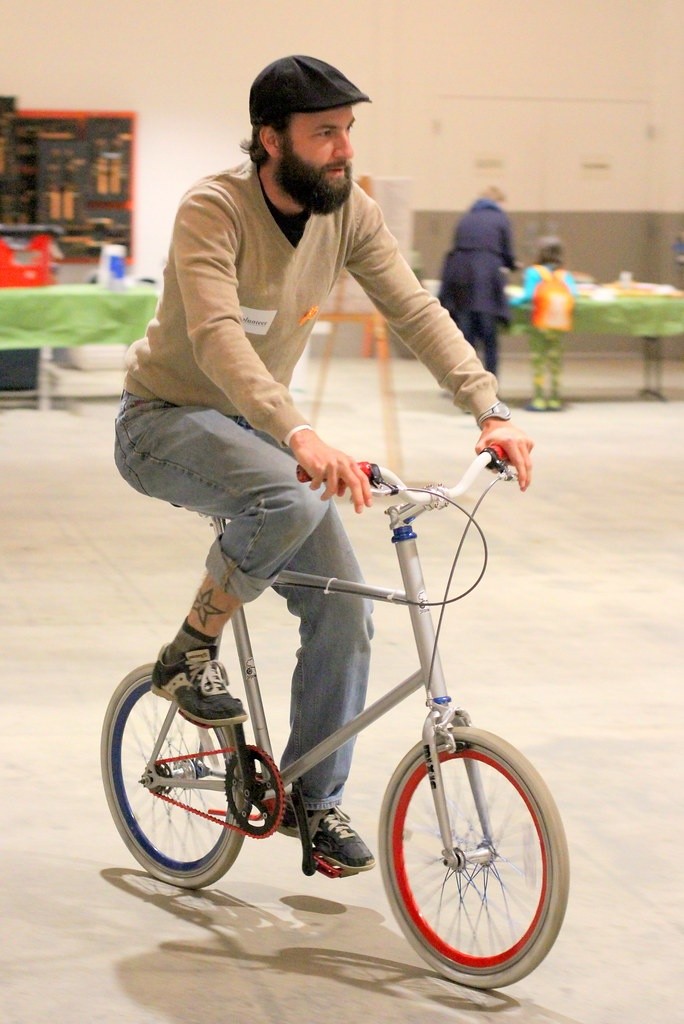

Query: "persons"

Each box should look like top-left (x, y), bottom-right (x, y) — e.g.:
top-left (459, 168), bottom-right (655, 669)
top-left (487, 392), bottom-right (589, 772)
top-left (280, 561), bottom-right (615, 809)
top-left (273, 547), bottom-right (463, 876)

top-left (115, 55), bottom-right (533, 872)
top-left (509, 237), bottom-right (576, 411)
top-left (440, 186), bottom-right (524, 378)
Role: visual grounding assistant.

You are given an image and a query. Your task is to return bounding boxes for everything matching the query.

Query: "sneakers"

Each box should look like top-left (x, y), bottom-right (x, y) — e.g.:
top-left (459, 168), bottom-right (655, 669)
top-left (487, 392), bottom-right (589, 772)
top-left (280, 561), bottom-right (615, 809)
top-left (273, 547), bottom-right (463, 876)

top-left (273, 800), bottom-right (375, 870)
top-left (151, 642), bottom-right (248, 725)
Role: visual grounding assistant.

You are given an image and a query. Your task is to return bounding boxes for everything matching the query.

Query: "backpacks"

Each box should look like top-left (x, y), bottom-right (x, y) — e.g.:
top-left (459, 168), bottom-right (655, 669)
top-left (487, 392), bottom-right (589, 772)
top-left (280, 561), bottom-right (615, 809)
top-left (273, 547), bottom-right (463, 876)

top-left (532, 264), bottom-right (574, 332)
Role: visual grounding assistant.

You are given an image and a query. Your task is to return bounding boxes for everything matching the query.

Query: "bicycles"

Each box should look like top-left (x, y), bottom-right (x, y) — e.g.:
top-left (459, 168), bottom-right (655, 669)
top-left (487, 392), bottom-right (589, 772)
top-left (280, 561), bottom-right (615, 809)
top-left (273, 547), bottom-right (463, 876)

top-left (99, 443), bottom-right (571, 993)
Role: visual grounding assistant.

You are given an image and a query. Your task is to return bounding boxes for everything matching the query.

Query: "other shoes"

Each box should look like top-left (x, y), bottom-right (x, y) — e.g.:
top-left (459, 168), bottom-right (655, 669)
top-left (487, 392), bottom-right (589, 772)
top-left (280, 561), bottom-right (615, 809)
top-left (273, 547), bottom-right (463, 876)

top-left (547, 398), bottom-right (561, 411)
top-left (526, 397), bottom-right (547, 411)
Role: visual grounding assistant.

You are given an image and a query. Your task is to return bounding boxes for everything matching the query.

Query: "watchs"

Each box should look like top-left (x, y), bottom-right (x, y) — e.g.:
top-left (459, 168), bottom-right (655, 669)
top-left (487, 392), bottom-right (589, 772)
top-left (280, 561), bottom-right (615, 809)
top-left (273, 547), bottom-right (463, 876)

top-left (476, 402), bottom-right (511, 426)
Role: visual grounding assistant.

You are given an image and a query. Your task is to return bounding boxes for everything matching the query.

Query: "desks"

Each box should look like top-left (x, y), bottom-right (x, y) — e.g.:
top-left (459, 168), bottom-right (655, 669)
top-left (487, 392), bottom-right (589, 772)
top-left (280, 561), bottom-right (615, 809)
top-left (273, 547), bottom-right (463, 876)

top-left (506, 280), bottom-right (684, 402)
top-left (0, 282), bottom-right (157, 411)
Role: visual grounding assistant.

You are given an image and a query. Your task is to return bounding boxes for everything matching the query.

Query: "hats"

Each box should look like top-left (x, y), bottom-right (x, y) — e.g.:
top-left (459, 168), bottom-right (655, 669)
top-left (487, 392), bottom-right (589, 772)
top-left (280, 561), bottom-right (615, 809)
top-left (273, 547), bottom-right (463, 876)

top-left (249, 55), bottom-right (369, 126)
